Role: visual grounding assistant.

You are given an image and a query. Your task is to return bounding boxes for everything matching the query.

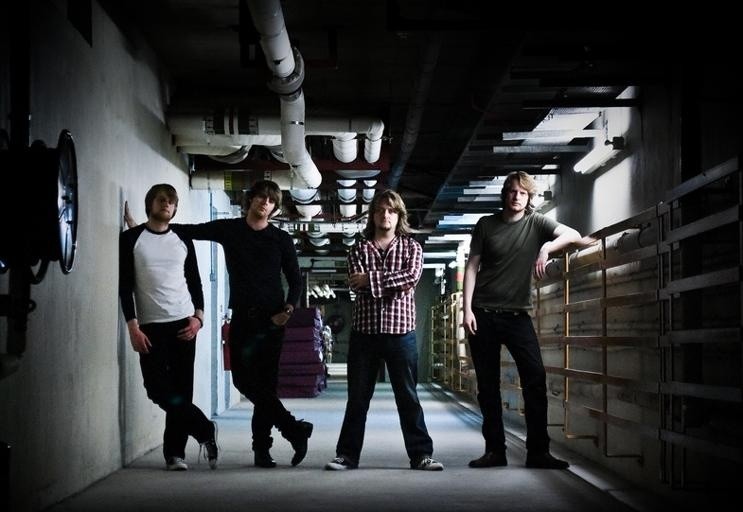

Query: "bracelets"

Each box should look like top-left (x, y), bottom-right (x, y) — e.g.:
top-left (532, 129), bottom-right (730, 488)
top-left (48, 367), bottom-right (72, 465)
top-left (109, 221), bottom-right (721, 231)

top-left (284, 307), bottom-right (293, 317)
top-left (193, 316), bottom-right (203, 328)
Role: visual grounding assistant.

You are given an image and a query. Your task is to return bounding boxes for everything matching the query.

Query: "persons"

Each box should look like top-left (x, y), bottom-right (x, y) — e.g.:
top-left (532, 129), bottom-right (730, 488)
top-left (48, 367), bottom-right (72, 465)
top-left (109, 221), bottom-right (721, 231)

top-left (463, 171), bottom-right (582, 470)
top-left (119, 184), bottom-right (222, 469)
top-left (325, 188), bottom-right (443, 471)
top-left (125, 181), bottom-right (313, 468)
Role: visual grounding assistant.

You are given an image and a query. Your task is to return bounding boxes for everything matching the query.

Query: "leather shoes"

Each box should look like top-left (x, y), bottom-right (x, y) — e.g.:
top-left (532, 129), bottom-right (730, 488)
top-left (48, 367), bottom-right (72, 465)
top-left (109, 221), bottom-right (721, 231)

top-left (526, 453), bottom-right (569, 469)
top-left (254, 454), bottom-right (276, 467)
top-left (469, 453), bottom-right (508, 467)
top-left (291, 422), bottom-right (313, 466)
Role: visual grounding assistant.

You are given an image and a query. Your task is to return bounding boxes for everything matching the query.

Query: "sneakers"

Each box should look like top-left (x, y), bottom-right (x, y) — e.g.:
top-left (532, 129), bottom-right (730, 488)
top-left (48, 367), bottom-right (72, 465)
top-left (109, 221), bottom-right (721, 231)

top-left (410, 455), bottom-right (444, 471)
top-left (326, 456), bottom-right (358, 470)
top-left (207, 421), bottom-right (218, 469)
top-left (164, 454), bottom-right (188, 472)
